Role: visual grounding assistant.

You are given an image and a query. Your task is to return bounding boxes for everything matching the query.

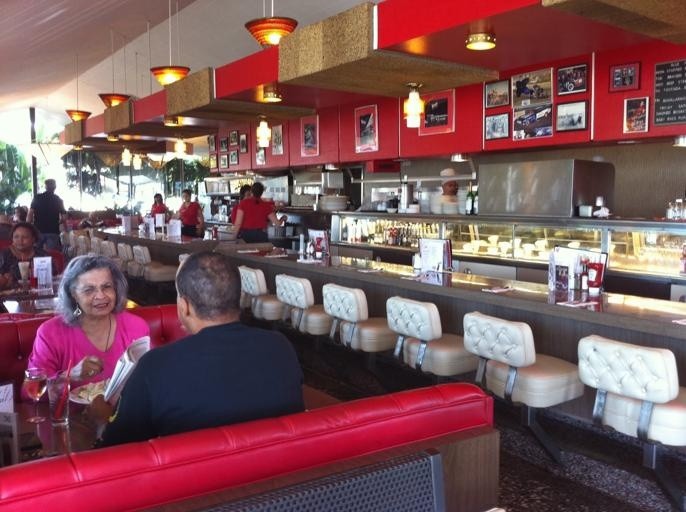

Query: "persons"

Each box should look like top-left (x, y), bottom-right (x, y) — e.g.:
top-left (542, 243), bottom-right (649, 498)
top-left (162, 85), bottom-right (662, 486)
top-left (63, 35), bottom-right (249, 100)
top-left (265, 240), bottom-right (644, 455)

top-left (231, 184), bottom-right (252, 225)
top-left (150, 193), bottom-right (171, 233)
top-left (233, 182), bottom-right (288, 243)
top-left (0, 179), bottom-right (67, 280)
top-left (19, 253), bottom-right (149, 401)
top-left (430, 166), bottom-right (459, 214)
top-left (177, 189), bottom-right (204, 238)
top-left (100, 250), bottom-right (303, 452)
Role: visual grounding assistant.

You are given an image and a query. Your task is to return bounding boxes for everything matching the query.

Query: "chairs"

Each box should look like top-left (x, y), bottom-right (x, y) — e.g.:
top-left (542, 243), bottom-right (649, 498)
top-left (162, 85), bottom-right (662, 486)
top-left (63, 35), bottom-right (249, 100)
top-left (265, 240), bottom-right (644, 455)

top-left (576, 333), bottom-right (686, 511)
top-left (462, 313), bottom-right (586, 466)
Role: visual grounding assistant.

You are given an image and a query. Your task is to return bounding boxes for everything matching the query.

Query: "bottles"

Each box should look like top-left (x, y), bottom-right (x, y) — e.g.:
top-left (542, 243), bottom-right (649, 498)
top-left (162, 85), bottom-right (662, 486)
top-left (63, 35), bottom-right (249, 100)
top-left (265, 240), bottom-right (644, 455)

top-left (666, 198), bottom-right (686, 220)
top-left (309, 238), bottom-right (315, 258)
top-left (315, 238), bottom-right (322, 258)
top-left (466, 181), bottom-right (475, 215)
top-left (681, 245), bottom-right (686, 273)
top-left (414, 253), bottom-right (421, 276)
top-left (582, 264), bottom-right (589, 291)
top-left (575, 255), bottom-right (583, 291)
top-left (36, 261), bottom-right (48, 296)
top-left (473, 182), bottom-right (478, 215)
top-left (343, 219), bottom-right (440, 248)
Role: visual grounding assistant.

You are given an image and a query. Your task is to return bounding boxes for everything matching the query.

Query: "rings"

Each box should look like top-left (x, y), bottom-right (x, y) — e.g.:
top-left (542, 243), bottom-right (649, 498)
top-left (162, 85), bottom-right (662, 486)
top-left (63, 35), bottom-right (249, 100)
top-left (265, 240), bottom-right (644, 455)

top-left (88, 369), bottom-right (97, 377)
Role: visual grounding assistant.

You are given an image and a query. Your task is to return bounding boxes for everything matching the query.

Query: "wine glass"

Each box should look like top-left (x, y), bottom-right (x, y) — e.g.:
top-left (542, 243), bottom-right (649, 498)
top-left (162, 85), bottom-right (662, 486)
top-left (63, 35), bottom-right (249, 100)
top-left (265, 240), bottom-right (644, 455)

top-left (24, 366), bottom-right (48, 422)
top-left (637, 242), bottom-right (683, 273)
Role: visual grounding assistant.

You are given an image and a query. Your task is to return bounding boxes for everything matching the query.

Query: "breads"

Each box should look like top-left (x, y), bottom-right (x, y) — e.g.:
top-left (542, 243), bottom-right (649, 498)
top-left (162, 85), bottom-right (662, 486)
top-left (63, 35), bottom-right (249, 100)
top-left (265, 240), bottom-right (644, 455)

top-left (79, 376), bottom-right (110, 401)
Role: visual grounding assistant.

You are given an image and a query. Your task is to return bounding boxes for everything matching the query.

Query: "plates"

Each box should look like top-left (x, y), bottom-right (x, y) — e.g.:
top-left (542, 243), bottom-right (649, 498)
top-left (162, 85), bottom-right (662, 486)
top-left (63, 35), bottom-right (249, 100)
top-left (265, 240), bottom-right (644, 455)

top-left (319, 196), bottom-right (349, 210)
top-left (421, 194), bottom-right (466, 214)
top-left (68, 382), bottom-right (103, 404)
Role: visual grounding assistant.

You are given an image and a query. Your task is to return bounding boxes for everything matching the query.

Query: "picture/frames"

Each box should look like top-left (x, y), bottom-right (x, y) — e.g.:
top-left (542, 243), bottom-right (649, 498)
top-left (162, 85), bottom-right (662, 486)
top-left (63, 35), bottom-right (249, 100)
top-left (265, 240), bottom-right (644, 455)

top-left (207, 119), bottom-right (284, 170)
top-left (418, 88), bottom-right (455, 135)
top-left (300, 114), bottom-right (320, 158)
top-left (354, 104), bottom-right (379, 154)
top-left (485, 58), bottom-right (649, 140)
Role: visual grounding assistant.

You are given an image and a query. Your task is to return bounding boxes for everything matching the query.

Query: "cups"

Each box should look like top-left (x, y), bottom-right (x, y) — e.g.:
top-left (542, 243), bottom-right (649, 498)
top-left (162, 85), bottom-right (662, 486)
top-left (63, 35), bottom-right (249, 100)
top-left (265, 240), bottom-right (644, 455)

top-left (409, 204), bottom-right (419, 213)
top-left (48, 375), bottom-right (69, 427)
top-left (3, 301), bottom-right (19, 313)
top-left (18, 262), bottom-right (29, 293)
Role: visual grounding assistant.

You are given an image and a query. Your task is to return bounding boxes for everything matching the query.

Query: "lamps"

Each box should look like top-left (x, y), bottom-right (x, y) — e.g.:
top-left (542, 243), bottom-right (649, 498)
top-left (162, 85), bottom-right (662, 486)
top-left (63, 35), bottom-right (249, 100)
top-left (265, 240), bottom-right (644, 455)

top-left (263, 92), bottom-right (282, 103)
top-left (465, 32), bottom-right (496, 51)
top-left (66, 1), bottom-right (191, 171)
top-left (404, 83), bottom-right (425, 129)
top-left (245, 0), bottom-right (298, 48)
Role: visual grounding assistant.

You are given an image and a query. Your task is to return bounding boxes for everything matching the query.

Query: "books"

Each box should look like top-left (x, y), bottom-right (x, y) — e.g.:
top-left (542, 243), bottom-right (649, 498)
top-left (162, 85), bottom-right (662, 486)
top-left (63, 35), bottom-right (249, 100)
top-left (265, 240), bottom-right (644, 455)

top-left (548, 291), bottom-right (607, 313)
top-left (308, 252), bottom-right (332, 267)
top-left (549, 244), bottom-right (609, 292)
top-left (104, 337), bottom-right (151, 403)
top-left (306, 229), bottom-right (328, 251)
top-left (420, 239), bottom-right (454, 272)
top-left (420, 271), bottom-right (453, 288)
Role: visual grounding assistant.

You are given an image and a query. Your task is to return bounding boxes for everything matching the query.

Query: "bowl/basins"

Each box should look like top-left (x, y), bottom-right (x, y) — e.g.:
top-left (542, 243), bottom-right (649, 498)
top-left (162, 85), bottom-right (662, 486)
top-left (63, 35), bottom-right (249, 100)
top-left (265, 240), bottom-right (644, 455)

top-left (387, 209), bottom-right (396, 213)
top-left (407, 208), bottom-right (418, 213)
